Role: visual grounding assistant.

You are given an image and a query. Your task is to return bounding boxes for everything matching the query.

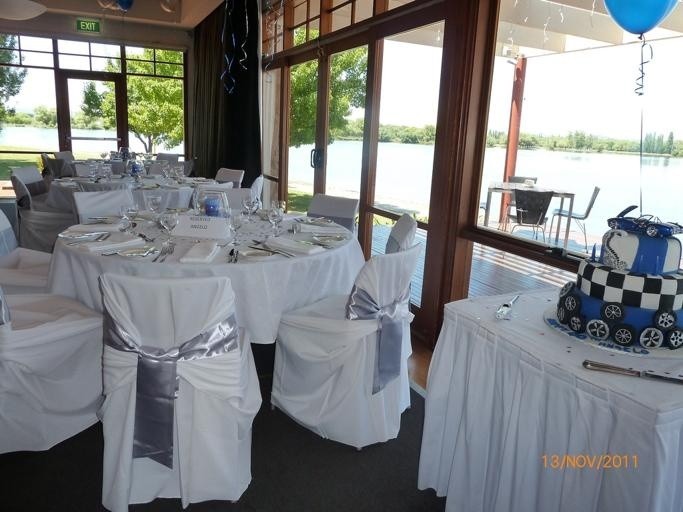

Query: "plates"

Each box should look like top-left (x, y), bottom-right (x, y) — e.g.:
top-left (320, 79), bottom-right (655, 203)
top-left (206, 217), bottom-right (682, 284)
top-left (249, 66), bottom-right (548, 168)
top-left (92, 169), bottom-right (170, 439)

top-left (54, 189), bottom-right (348, 263)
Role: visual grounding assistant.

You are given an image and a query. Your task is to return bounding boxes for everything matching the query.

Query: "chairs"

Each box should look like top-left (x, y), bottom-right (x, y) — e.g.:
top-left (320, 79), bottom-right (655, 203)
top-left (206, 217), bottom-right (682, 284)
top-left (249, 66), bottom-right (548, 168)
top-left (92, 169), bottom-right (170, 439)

top-left (498, 188), bottom-right (554, 243)
top-left (0, 209), bottom-right (52, 294)
top-left (95, 273), bottom-right (264, 512)
top-left (549, 186), bottom-right (600, 254)
top-left (270, 242), bottom-right (423, 452)
top-left (11, 151), bottom-right (263, 253)
top-left (498, 176), bottom-right (537, 231)
top-left (479, 202), bottom-right (485, 210)
top-left (0, 286), bottom-right (104, 454)
top-left (385, 213), bottom-right (418, 254)
top-left (307, 193), bottom-right (359, 235)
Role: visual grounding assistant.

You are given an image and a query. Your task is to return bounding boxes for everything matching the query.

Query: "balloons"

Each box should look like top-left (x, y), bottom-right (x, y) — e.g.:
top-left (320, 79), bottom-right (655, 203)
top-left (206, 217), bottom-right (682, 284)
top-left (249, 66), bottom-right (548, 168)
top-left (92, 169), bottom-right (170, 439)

top-left (603, 1), bottom-right (681, 40)
top-left (159, 1), bottom-right (176, 15)
top-left (98, 0), bottom-right (133, 14)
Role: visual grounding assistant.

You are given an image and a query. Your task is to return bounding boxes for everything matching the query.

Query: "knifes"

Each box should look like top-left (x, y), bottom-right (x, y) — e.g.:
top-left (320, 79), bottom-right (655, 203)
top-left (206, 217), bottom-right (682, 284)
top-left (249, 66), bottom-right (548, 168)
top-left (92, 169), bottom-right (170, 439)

top-left (582, 357), bottom-right (682, 386)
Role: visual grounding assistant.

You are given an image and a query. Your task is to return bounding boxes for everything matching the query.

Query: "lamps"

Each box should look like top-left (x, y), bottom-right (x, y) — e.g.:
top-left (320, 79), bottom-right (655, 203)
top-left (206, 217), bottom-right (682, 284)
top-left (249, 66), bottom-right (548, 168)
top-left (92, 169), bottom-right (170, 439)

top-left (0, 0), bottom-right (48, 20)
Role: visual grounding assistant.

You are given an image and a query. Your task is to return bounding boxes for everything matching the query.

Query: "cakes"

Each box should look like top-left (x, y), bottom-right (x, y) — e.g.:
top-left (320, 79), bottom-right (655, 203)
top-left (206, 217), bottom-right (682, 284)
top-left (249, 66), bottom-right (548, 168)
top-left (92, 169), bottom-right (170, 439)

top-left (556, 206), bottom-right (683, 351)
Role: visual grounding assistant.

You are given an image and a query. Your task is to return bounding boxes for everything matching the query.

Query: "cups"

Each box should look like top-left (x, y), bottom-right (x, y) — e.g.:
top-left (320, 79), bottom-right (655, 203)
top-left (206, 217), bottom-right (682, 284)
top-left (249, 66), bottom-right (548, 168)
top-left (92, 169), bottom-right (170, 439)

top-left (526, 179), bottom-right (535, 186)
top-left (54, 149), bottom-right (218, 188)
